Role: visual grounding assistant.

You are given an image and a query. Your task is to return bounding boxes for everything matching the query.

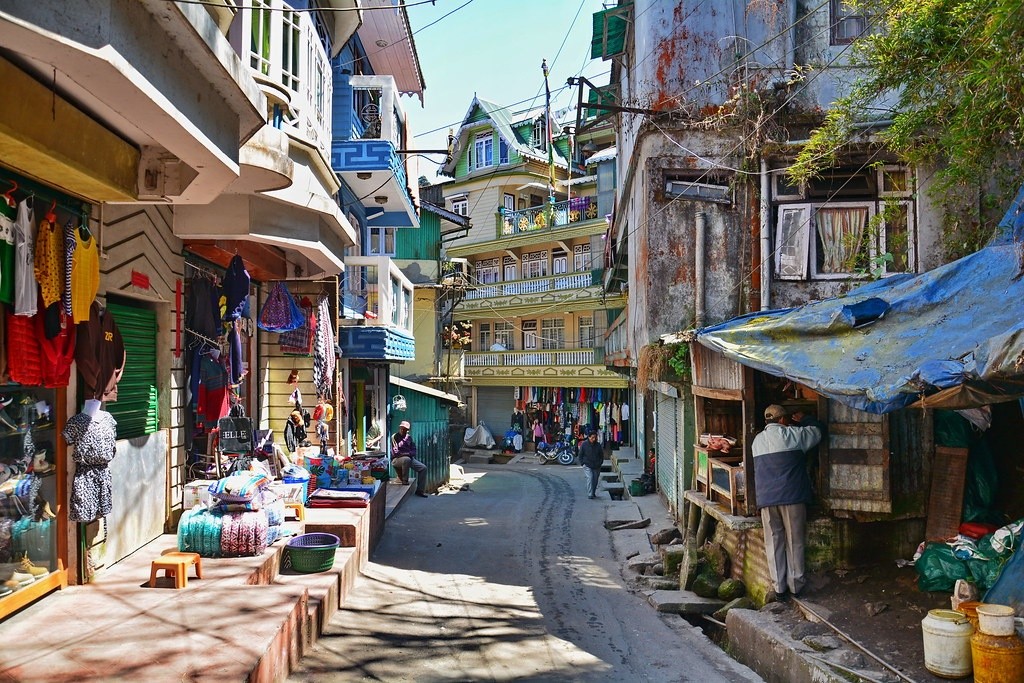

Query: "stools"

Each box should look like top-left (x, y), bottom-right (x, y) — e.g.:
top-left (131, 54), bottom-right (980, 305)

top-left (284, 498), bottom-right (305, 522)
top-left (149, 552), bottom-right (203, 590)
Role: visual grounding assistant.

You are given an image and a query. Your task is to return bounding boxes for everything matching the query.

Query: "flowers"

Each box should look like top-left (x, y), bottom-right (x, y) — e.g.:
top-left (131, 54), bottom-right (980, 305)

top-left (437, 321), bottom-right (471, 348)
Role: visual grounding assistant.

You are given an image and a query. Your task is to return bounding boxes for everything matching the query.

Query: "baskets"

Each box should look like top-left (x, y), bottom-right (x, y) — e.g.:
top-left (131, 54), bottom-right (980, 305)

top-left (286, 533), bottom-right (339, 572)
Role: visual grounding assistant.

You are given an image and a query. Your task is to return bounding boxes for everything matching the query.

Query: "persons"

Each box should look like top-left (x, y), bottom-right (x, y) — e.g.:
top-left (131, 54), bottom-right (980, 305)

top-left (532, 418), bottom-right (544, 457)
top-left (391, 421), bottom-right (429, 498)
top-left (641, 447), bottom-right (655, 484)
top-left (752, 405), bottom-right (822, 599)
top-left (578, 432), bottom-right (604, 499)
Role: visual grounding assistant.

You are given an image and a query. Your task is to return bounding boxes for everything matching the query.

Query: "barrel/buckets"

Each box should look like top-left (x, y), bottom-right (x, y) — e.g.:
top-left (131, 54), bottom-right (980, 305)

top-left (957, 602), bottom-right (983, 631)
top-left (970, 628), bottom-right (1024, 683)
top-left (976, 603), bottom-right (1016, 635)
top-left (921, 609), bottom-right (972, 678)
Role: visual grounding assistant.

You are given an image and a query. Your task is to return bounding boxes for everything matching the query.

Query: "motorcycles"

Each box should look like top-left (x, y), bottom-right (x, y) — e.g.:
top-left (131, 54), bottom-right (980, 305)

top-left (534, 434), bottom-right (575, 465)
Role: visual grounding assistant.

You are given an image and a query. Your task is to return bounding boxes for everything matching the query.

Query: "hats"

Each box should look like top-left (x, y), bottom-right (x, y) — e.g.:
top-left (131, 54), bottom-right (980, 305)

top-left (399, 420), bottom-right (410, 430)
top-left (764, 405), bottom-right (788, 420)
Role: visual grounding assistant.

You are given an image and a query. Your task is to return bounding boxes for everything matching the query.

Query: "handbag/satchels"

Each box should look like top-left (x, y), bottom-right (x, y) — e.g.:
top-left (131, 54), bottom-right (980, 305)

top-left (258, 282), bottom-right (305, 333)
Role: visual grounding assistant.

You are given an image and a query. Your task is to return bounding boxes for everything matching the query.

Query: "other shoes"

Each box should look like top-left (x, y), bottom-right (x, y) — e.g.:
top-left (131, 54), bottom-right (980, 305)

top-left (775, 590), bottom-right (789, 601)
top-left (791, 583), bottom-right (808, 599)
top-left (402, 480), bottom-right (409, 485)
top-left (0, 392), bottom-right (57, 597)
top-left (415, 491), bottom-right (428, 498)
top-left (589, 494), bottom-right (596, 499)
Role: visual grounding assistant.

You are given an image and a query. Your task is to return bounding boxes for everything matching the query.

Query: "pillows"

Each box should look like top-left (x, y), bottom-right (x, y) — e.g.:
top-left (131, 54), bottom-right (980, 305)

top-left (210, 492), bottom-right (283, 512)
top-left (208, 468), bottom-right (270, 501)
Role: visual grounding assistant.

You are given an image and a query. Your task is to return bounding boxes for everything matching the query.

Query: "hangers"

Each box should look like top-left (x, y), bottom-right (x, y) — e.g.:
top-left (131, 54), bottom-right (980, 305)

top-left (66, 210), bottom-right (73, 224)
top-left (187, 332), bottom-right (222, 362)
top-left (41, 199), bottom-right (57, 224)
top-left (192, 247), bottom-right (239, 282)
top-left (79, 211), bottom-right (92, 236)
top-left (25, 189), bottom-right (36, 209)
top-left (95, 298), bottom-right (104, 317)
top-left (4, 179), bottom-right (18, 207)
